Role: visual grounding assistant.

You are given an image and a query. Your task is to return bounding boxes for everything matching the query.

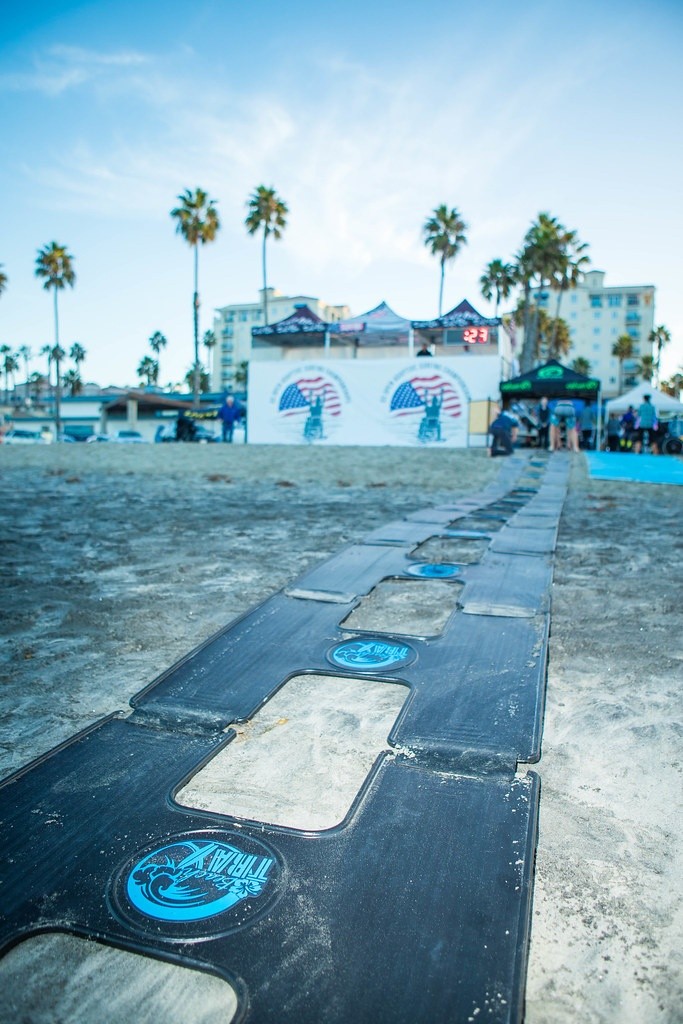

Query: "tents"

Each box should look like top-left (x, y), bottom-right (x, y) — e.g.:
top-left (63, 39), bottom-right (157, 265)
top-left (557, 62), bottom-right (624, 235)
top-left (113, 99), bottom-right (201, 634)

top-left (604, 378), bottom-right (683, 439)
top-left (252, 299), bottom-right (502, 356)
top-left (500, 358), bottom-right (602, 452)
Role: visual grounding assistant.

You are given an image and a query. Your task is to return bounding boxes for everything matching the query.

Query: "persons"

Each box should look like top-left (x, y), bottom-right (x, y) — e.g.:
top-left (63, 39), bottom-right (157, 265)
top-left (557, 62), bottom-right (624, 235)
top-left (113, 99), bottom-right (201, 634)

top-left (417, 344), bottom-right (432, 356)
top-left (487, 397), bottom-right (597, 457)
top-left (217, 396), bottom-right (240, 443)
top-left (607, 395), bottom-right (683, 455)
